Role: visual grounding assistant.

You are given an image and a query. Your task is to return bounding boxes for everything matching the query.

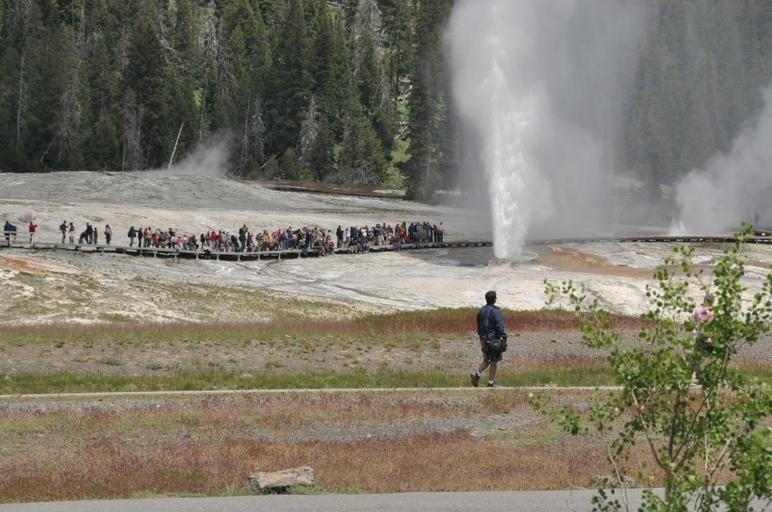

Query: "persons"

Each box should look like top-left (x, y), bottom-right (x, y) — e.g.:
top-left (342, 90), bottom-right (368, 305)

top-left (4, 220), bottom-right (17, 240)
top-left (29, 222), bottom-right (37, 243)
top-left (470, 291), bottom-right (508, 387)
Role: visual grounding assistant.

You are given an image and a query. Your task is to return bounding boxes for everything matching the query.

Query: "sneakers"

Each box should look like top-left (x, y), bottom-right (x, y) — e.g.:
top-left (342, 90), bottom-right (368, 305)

top-left (470, 372), bottom-right (479, 387)
top-left (487, 381), bottom-right (499, 387)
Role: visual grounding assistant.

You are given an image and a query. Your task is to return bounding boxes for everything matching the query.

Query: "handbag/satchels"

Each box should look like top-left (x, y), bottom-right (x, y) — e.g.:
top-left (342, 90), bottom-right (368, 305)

top-left (483, 337), bottom-right (508, 353)
